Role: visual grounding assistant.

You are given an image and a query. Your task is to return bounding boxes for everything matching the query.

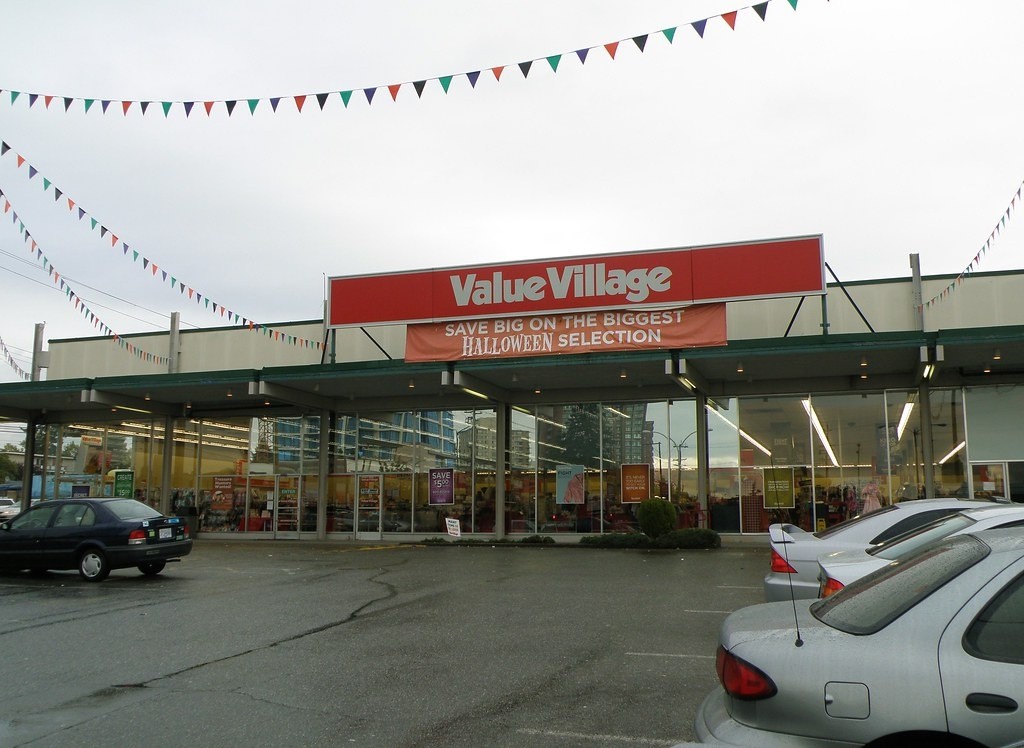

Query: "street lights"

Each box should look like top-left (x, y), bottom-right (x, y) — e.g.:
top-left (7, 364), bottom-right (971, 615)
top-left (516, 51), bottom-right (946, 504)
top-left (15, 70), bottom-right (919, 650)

top-left (642, 427), bottom-right (714, 493)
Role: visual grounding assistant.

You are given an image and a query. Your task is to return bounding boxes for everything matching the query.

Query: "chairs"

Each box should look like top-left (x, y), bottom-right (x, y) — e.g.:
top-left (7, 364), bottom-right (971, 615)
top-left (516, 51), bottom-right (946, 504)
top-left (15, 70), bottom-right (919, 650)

top-left (61, 511), bottom-right (76, 525)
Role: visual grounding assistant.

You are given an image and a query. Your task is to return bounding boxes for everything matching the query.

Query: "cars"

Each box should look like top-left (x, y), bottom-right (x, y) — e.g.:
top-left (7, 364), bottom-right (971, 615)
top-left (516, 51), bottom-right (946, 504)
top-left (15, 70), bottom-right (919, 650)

top-left (541, 516), bottom-right (641, 534)
top-left (762, 496), bottom-right (1019, 603)
top-left (0, 498), bottom-right (48, 523)
top-left (817, 501), bottom-right (1024, 600)
top-left (0, 498), bottom-right (16, 515)
top-left (0, 497), bottom-right (194, 583)
top-left (695, 526), bottom-right (1024, 747)
top-left (491, 519), bottom-right (536, 532)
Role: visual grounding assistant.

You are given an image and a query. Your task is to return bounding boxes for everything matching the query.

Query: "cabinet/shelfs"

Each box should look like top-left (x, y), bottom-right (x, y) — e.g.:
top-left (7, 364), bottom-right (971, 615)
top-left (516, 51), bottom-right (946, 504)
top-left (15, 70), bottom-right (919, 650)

top-left (133, 480), bottom-right (886, 531)
